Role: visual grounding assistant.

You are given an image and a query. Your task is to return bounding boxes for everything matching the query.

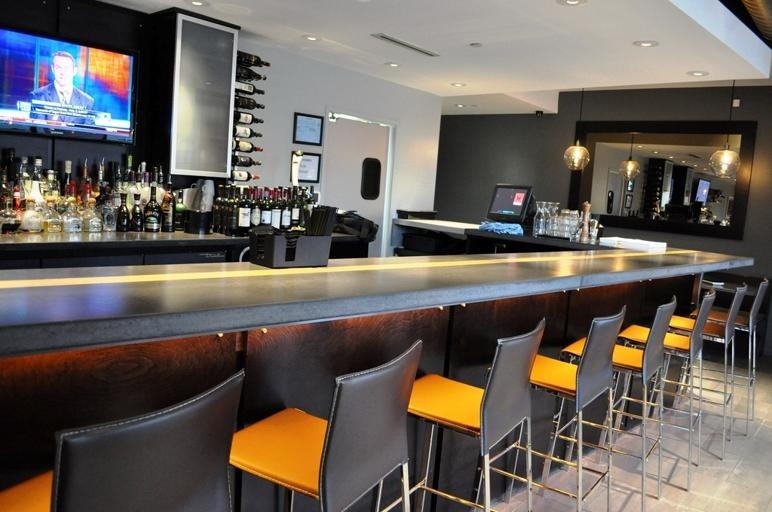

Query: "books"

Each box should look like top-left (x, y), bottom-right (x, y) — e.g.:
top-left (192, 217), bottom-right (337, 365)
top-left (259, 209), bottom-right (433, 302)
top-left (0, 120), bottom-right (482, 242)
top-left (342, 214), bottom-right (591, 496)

top-left (599, 236), bottom-right (666, 252)
top-left (703, 278), bottom-right (725, 285)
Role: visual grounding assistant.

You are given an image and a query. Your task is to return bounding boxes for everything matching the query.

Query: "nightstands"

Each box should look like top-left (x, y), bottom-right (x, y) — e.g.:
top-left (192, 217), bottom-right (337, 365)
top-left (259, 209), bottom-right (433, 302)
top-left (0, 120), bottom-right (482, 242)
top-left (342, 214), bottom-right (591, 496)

top-left (406, 315), bottom-right (546, 512)
top-left (670, 281), bottom-right (754, 399)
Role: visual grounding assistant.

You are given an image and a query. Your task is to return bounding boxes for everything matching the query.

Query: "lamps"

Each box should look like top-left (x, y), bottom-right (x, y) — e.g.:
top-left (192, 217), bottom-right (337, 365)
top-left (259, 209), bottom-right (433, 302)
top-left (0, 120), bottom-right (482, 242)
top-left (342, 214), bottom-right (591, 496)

top-left (617, 134), bottom-right (640, 183)
top-left (707, 79), bottom-right (741, 181)
top-left (563, 88), bottom-right (591, 171)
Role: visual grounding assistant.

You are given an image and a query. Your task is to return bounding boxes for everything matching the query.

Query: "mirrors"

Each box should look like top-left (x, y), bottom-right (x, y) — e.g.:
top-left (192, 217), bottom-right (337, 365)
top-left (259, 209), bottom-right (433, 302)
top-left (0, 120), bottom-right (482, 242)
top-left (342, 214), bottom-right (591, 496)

top-left (564, 121), bottom-right (757, 241)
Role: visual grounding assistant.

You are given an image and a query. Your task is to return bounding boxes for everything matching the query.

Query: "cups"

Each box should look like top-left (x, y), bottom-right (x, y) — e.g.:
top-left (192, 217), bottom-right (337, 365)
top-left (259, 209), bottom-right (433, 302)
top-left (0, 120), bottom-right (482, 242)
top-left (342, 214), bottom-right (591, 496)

top-left (191, 176), bottom-right (215, 213)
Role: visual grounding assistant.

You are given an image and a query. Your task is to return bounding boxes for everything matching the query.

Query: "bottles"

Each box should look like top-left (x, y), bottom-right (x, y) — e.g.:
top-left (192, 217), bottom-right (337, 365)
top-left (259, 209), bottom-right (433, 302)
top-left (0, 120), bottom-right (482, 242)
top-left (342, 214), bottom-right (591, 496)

top-left (0, 148), bottom-right (187, 234)
top-left (211, 184), bottom-right (315, 237)
top-left (230, 50), bottom-right (270, 182)
top-left (533, 201), bottom-right (602, 244)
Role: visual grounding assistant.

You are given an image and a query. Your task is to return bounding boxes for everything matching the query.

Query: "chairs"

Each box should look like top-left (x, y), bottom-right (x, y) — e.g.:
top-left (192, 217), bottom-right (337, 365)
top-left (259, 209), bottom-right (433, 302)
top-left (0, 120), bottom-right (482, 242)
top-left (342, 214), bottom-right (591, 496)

top-left (485, 303), bottom-right (627, 471)
top-left (228, 339), bottom-right (423, 512)
top-left (1, 368), bottom-right (245, 511)
top-left (618, 286), bottom-right (716, 432)
top-left (693, 278), bottom-right (770, 387)
top-left (559, 294), bottom-right (677, 471)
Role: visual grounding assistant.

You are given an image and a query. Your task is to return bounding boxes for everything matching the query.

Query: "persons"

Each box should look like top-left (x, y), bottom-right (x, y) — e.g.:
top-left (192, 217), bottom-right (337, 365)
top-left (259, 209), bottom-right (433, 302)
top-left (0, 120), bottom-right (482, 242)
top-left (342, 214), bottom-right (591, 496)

top-left (28, 51), bottom-right (95, 125)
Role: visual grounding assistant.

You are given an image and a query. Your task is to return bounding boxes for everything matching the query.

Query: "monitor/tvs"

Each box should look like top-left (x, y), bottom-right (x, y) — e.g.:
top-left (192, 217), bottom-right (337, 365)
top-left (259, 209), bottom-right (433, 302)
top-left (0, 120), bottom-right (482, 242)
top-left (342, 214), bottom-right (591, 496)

top-left (487, 182), bottom-right (534, 222)
top-left (694, 178), bottom-right (711, 206)
top-left (0, 28), bottom-right (137, 146)
